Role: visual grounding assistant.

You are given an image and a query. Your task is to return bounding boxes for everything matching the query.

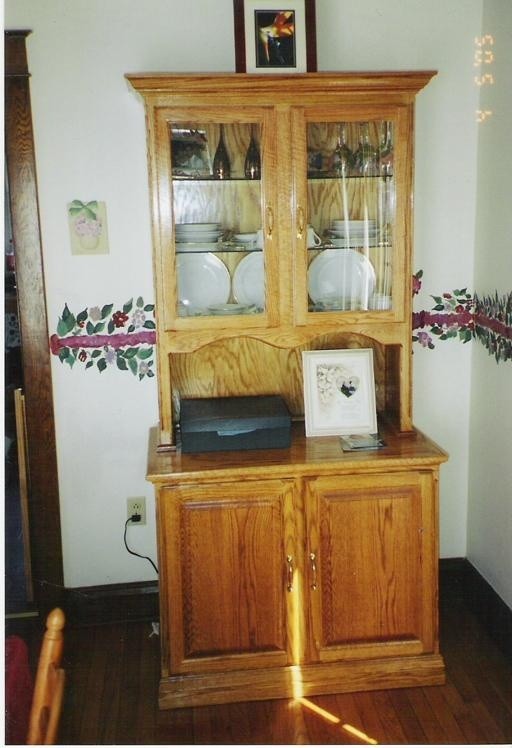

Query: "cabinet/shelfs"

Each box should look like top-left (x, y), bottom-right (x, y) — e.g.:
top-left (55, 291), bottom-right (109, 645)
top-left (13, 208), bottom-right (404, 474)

top-left (125, 70), bottom-right (452, 712)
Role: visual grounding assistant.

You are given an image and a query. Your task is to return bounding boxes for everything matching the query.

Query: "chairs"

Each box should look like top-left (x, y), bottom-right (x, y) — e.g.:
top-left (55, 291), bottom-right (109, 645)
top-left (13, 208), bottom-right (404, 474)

top-left (25, 607), bottom-right (71, 744)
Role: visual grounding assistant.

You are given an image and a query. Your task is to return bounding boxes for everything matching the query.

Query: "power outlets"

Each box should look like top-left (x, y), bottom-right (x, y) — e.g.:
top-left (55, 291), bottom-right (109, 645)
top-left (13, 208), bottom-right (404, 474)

top-left (126, 495), bottom-right (145, 526)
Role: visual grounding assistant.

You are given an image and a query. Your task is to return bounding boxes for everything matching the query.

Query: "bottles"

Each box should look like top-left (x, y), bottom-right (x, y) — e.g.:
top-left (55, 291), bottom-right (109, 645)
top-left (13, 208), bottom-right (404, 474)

top-left (332, 121), bottom-right (394, 178)
top-left (213, 123), bottom-right (261, 180)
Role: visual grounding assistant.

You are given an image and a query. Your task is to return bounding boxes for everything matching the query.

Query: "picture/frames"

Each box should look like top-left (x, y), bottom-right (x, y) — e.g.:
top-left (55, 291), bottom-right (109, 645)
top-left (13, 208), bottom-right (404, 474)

top-left (301, 346), bottom-right (379, 438)
top-left (231, 0), bottom-right (319, 73)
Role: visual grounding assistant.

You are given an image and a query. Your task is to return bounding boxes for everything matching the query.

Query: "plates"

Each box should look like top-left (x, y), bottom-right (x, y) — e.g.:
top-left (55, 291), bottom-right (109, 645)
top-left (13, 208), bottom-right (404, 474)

top-left (174, 220), bottom-right (378, 315)
